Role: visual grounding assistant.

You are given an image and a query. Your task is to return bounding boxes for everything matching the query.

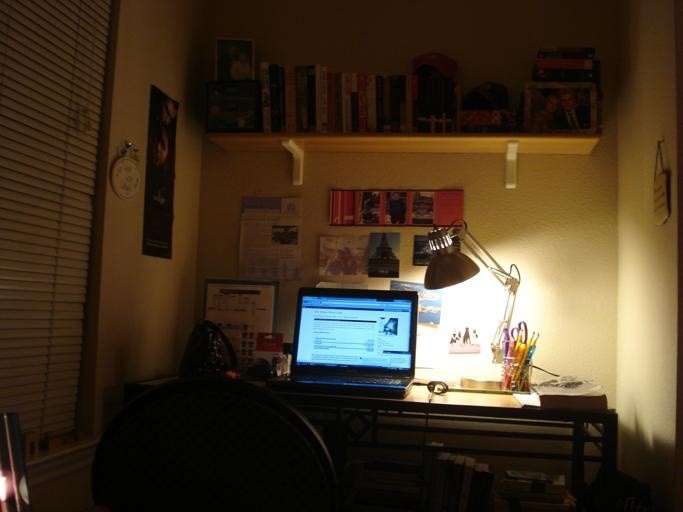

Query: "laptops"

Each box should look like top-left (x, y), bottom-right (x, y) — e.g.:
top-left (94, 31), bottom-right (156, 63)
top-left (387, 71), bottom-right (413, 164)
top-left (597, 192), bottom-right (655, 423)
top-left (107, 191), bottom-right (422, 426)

top-left (266, 286), bottom-right (419, 399)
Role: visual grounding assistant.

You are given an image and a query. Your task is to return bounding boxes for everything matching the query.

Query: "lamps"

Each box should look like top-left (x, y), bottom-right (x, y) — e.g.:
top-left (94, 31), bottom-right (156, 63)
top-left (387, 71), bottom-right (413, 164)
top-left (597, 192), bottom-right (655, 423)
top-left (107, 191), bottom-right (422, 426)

top-left (424, 219), bottom-right (520, 365)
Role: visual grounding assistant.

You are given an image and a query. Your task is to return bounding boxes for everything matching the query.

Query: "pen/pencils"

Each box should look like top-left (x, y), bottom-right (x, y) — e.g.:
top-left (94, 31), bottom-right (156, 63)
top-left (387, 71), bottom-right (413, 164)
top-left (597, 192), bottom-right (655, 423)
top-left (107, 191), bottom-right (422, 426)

top-left (503, 328), bottom-right (539, 392)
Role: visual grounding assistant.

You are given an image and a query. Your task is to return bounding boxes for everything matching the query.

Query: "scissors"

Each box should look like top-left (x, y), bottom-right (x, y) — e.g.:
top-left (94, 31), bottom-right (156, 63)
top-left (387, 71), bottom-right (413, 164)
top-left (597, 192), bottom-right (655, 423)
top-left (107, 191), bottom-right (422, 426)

top-left (511, 321), bottom-right (527, 344)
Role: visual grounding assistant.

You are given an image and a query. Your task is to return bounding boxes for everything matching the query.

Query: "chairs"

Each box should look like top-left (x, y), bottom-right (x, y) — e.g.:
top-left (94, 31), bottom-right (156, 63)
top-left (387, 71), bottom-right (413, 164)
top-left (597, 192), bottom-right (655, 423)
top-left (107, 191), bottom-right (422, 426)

top-left (92, 379), bottom-right (338, 512)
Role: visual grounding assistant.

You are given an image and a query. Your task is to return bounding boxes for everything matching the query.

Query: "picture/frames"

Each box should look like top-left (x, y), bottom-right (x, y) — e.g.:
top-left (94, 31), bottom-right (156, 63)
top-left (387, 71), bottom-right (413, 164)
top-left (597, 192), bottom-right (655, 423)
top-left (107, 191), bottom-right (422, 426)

top-left (211, 35), bottom-right (261, 80)
top-left (204, 279), bottom-right (279, 372)
top-left (525, 82), bottom-right (597, 136)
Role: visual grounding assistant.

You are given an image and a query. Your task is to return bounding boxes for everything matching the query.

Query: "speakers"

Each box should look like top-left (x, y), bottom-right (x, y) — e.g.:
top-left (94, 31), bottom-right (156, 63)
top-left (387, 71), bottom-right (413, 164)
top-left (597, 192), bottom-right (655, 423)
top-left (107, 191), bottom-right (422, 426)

top-left (205, 79), bottom-right (263, 133)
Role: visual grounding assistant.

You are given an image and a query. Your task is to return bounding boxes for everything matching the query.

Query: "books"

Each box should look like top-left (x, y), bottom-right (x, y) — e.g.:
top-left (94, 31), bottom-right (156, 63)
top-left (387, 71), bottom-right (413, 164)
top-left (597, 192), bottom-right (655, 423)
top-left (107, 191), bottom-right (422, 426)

top-left (527, 375), bottom-right (608, 411)
top-left (258, 60), bottom-right (451, 134)
top-left (419, 437), bottom-right (576, 512)
top-left (211, 34), bottom-right (256, 83)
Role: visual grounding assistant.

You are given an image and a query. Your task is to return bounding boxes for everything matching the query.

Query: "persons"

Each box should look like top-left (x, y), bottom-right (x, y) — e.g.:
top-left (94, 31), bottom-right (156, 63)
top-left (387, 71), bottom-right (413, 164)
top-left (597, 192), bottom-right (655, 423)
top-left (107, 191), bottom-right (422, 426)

top-left (150, 93), bottom-right (177, 207)
top-left (386, 192), bottom-right (406, 225)
top-left (228, 51), bottom-right (250, 82)
top-left (326, 246), bottom-right (356, 275)
top-left (530, 92), bottom-right (560, 129)
top-left (553, 89), bottom-right (588, 129)
top-left (324, 248), bottom-right (344, 275)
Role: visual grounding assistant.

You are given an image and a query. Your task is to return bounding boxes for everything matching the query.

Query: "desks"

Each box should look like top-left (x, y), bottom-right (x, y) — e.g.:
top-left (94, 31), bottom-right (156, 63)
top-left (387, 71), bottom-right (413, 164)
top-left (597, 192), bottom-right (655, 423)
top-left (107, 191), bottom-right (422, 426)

top-left (125, 377), bottom-right (618, 512)
